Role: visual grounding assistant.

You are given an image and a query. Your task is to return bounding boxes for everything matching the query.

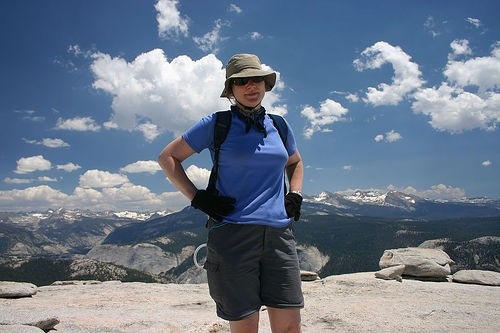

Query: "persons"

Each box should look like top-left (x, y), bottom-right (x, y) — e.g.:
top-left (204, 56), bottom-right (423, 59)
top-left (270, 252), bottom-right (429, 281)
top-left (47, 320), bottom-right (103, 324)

top-left (158, 54), bottom-right (303, 333)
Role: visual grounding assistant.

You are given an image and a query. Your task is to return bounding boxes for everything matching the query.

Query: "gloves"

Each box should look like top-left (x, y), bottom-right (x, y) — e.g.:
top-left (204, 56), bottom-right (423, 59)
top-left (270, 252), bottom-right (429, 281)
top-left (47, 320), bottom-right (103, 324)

top-left (191, 189), bottom-right (236, 222)
top-left (284, 192), bottom-right (303, 222)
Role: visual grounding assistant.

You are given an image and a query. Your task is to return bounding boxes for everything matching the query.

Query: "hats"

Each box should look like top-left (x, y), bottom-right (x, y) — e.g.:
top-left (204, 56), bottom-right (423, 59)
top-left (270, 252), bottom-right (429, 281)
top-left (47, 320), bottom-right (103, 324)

top-left (220, 53), bottom-right (276, 97)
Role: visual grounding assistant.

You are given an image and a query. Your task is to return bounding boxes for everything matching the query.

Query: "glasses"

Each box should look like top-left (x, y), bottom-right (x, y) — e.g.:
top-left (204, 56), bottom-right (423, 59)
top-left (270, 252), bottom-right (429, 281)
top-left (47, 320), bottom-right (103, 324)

top-left (231, 76), bottom-right (265, 86)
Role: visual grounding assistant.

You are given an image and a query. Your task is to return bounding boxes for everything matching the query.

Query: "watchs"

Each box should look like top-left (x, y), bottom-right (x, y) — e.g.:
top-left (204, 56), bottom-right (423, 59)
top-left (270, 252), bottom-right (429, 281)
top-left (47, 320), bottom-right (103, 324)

top-left (289, 191), bottom-right (302, 195)
